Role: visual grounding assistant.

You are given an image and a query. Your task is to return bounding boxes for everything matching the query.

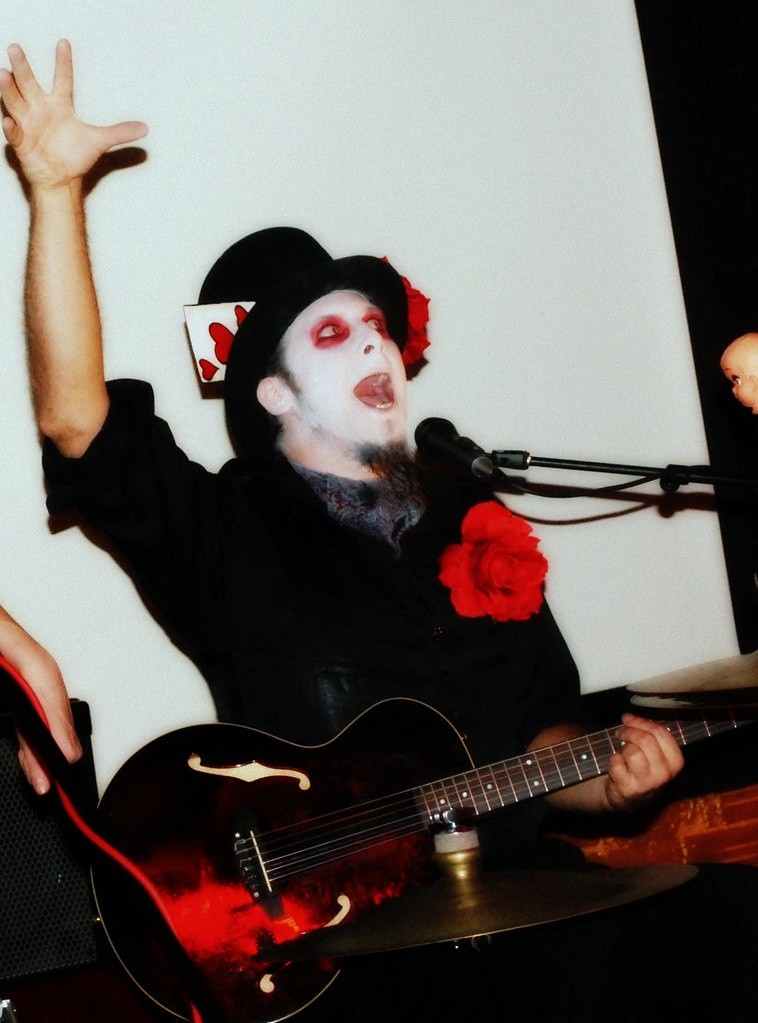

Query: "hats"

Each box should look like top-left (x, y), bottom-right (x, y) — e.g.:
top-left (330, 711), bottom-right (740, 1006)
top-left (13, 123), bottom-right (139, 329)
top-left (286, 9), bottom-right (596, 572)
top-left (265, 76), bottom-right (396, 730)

top-left (183, 229), bottom-right (429, 466)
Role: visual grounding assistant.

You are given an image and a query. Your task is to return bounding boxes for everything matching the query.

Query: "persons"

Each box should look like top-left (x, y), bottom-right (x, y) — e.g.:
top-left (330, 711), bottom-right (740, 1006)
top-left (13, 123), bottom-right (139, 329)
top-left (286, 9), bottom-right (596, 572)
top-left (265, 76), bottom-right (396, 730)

top-left (0, 605), bottom-right (81, 794)
top-left (1, 38), bottom-right (682, 837)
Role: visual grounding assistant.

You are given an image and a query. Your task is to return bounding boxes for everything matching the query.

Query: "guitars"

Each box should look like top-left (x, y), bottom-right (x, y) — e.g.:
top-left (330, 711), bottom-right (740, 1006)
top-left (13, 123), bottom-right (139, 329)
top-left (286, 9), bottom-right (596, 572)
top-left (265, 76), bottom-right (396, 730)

top-left (83, 694), bottom-right (758, 1023)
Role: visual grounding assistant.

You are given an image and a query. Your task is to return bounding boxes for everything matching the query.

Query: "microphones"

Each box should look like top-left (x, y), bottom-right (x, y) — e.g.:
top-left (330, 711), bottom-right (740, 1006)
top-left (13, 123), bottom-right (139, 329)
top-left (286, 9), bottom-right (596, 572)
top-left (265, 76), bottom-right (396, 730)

top-left (415, 417), bottom-right (507, 483)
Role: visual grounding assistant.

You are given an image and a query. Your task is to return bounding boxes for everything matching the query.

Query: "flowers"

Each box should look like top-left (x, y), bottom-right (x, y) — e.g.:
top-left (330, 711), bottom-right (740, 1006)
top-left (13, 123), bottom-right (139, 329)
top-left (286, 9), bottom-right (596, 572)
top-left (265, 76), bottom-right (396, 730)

top-left (437, 501), bottom-right (550, 622)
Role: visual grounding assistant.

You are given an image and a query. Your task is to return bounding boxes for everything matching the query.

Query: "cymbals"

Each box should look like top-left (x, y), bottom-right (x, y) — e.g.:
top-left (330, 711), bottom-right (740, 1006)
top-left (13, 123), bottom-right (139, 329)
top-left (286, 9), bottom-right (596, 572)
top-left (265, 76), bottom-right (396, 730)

top-left (315, 849), bottom-right (701, 960)
